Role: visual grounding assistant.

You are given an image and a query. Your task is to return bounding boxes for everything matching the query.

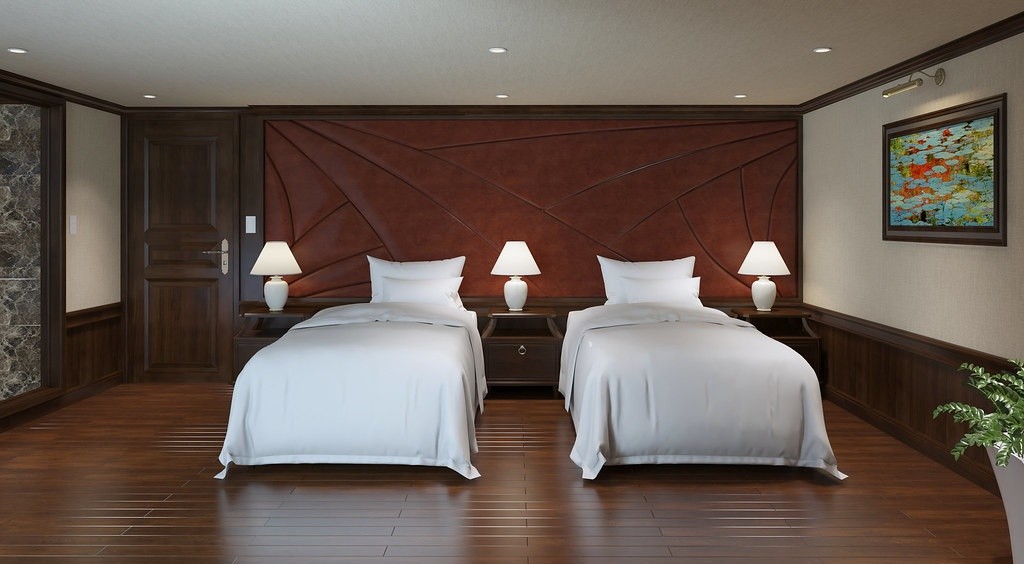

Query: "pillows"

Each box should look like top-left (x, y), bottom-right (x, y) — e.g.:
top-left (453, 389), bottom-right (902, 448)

top-left (367, 254), bottom-right (467, 311)
top-left (596, 253), bottom-right (704, 307)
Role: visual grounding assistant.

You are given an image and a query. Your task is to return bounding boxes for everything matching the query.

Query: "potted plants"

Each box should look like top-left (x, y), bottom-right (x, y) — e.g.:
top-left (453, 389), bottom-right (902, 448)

top-left (932, 357), bottom-right (1024, 564)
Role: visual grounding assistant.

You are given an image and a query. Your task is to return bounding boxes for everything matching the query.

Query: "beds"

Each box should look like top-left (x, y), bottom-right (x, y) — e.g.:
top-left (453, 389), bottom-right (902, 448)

top-left (558, 302), bottom-right (850, 481)
top-left (214, 303), bottom-right (488, 480)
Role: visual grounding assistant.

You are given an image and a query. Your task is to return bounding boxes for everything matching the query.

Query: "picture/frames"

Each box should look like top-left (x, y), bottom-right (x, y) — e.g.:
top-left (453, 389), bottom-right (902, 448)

top-left (881, 91), bottom-right (1008, 246)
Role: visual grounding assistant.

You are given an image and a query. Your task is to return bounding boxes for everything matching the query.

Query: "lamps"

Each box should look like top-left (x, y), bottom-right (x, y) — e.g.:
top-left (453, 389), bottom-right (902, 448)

top-left (489, 240), bottom-right (542, 311)
top-left (737, 241), bottom-right (791, 312)
top-left (248, 240), bottom-right (302, 311)
top-left (882, 68), bottom-right (946, 99)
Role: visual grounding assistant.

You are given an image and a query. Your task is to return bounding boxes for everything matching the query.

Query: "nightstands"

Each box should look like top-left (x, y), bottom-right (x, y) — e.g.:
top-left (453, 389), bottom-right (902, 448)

top-left (480, 306), bottom-right (564, 399)
top-left (730, 307), bottom-right (824, 397)
top-left (232, 300), bottom-right (321, 388)
